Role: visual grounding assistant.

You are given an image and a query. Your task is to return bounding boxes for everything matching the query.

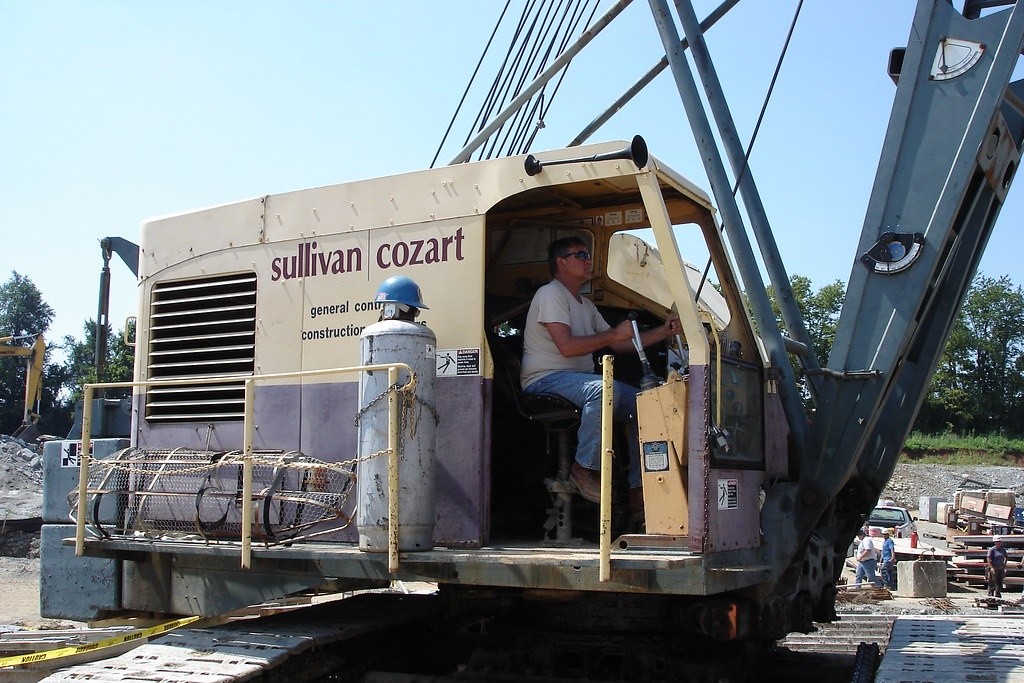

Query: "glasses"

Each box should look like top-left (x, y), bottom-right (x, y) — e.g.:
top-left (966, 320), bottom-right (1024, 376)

top-left (882, 533), bottom-right (888, 535)
top-left (564, 250), bottom-right (591, 260)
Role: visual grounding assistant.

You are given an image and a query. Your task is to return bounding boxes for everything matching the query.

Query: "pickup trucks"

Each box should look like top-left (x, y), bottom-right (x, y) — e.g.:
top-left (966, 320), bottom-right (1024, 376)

top-left (854, 506), bottom-right (917, 549)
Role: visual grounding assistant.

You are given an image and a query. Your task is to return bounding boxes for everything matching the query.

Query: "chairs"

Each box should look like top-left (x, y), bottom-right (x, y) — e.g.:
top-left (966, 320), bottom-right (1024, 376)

top-left (503, 347), bottom-right (593, 548)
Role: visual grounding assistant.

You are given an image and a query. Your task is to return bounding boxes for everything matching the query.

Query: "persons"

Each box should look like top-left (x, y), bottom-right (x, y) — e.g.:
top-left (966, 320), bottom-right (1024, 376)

top-left (878, 529), bottom-right (895, 589)
top-left (854, 529), bottom-right (884, 589)
top-left (987, 534), bottom-right (1008, 598)
top-left (1019, 555), bottom-right (1024, 598)
top-left (520, 236), bottom-right (685, 515)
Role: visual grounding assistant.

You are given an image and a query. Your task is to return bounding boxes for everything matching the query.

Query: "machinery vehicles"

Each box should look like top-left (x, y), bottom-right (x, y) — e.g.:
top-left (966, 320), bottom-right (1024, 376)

top-left (68, 0), bottom-right (1024, 649)
top-left (0, 332), bottom-right (46, 445)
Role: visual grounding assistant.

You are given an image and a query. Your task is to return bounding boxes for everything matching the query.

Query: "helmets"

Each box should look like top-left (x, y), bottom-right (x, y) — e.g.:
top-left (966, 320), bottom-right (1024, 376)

top-left (993, 535), bottom-right (1001, 541)
top-left (881, 528), bottom-right (890, 534)
top-left (375, 275), bottom-right (430, 310)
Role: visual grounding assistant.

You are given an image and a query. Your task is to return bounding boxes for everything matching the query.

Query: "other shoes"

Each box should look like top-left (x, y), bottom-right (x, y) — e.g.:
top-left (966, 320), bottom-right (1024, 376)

top-left (569, 461), bottom-right (602, 503)
top-left (629, 488), bottom-right (645, 516)
